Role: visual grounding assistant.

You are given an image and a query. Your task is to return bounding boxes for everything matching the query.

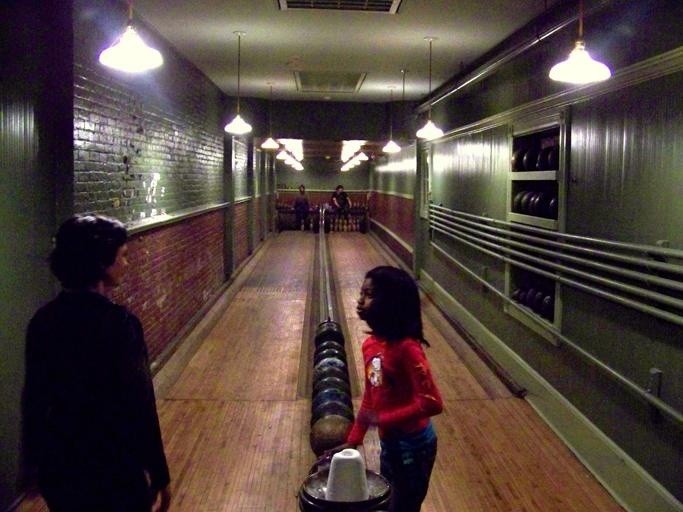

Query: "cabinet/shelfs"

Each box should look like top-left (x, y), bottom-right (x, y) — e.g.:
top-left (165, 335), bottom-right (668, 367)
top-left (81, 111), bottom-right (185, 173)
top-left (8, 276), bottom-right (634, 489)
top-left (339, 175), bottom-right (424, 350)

top-left (498, 103), bottom-right (570, 344)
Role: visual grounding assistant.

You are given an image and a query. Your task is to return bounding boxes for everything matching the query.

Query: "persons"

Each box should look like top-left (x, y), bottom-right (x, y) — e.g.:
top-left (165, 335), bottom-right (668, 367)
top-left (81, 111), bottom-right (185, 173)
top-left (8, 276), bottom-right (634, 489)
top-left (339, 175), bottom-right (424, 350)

top-left (19, 213), bottom-right (171, 512)
top-left (292, 184), bottom-right (312, 219)
top-left (331, 185), bottom-right (352, 209)
top-left (314, 266), bottom-right (443, 512)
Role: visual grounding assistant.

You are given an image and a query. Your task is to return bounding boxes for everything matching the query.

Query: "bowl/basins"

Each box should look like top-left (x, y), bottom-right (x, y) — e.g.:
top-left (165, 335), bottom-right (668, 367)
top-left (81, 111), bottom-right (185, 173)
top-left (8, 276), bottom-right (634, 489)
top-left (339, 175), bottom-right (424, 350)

top-left (299, 468), bottom-right (394, 511)
